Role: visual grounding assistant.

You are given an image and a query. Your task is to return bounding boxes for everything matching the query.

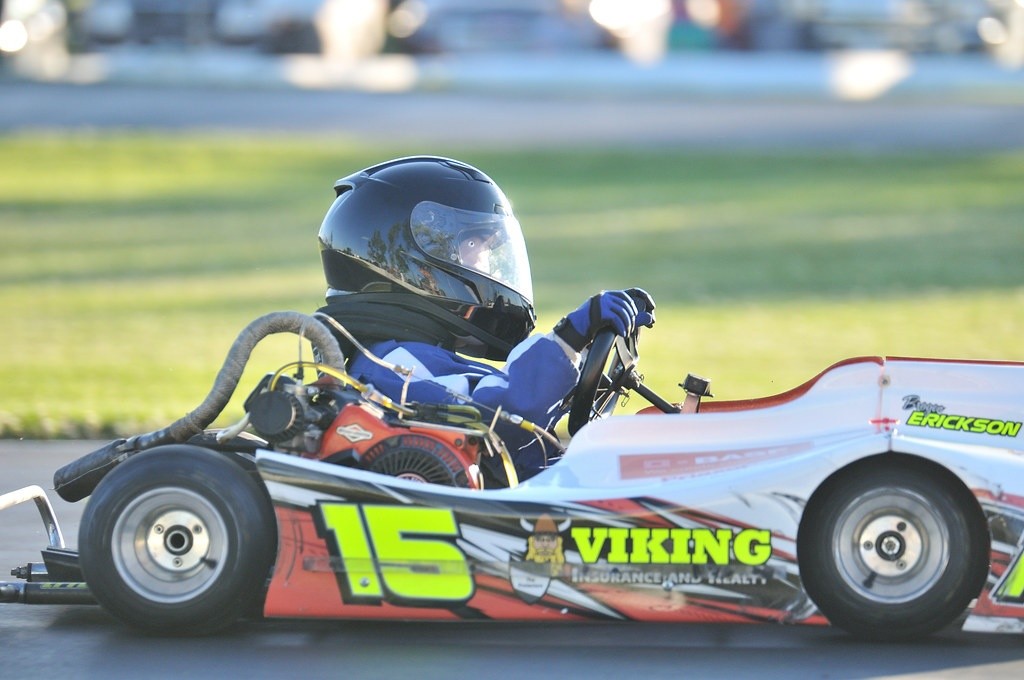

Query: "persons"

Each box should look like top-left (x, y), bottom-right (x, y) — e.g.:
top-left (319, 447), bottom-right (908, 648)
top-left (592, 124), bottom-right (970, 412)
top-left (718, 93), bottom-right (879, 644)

top-left (310, 155), bottom-right (656, 486)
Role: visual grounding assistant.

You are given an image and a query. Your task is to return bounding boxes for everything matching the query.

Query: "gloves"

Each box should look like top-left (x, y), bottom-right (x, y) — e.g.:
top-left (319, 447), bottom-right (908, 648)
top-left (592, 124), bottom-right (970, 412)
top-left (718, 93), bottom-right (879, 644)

top-left (554, 291), bottom-right (639, 353)
top-left (623, 287), bottom-right (656, 328)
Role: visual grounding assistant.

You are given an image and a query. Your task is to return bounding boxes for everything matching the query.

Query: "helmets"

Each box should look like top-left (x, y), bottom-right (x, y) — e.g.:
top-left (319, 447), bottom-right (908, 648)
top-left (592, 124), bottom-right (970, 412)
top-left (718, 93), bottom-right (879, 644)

top-left (318, 155), bottom-right (536, 360)
